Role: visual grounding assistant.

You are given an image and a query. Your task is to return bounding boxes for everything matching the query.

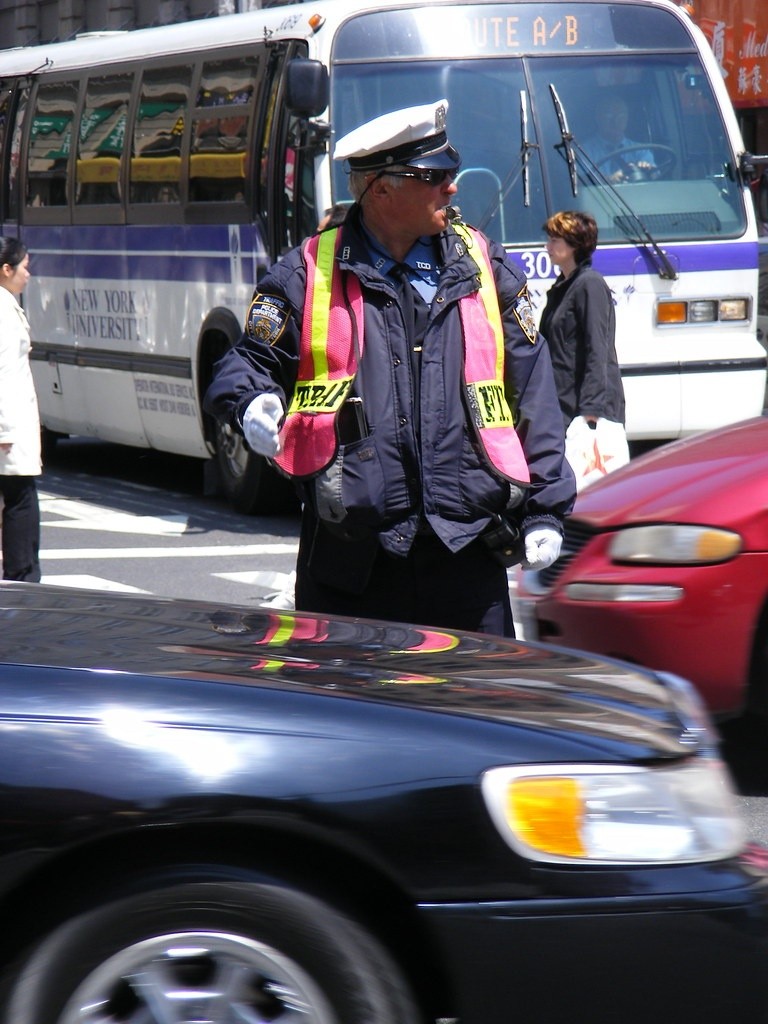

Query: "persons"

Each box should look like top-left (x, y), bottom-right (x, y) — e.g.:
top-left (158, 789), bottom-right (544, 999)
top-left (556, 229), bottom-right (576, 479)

top-left (0, 95), bottom-right (660, 637)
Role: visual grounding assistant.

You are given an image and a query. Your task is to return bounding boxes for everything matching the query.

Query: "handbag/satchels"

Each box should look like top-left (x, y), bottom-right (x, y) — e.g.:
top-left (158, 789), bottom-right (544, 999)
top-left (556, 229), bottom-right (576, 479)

top-left (559, 414), bottom-right (630, 493)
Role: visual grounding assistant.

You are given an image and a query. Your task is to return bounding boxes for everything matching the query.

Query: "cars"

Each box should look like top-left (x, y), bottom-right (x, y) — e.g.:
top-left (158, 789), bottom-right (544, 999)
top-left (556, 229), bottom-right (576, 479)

top-left (0, 583), bottom-right (767, 1024)
top-left (489, 416), bottom-right (767, 795)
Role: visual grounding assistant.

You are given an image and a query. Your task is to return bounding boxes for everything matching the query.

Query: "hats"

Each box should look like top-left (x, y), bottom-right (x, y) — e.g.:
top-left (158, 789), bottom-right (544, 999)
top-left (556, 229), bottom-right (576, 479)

top-left (333, 100), bottom-right (463, 170)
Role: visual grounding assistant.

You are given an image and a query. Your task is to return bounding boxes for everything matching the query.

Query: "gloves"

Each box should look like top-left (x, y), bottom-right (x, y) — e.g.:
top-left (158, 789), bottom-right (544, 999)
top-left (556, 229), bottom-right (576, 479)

top-left (242, 393), bottom-right (285, 459)
top-left (520, 522), bottom-right (565, 571)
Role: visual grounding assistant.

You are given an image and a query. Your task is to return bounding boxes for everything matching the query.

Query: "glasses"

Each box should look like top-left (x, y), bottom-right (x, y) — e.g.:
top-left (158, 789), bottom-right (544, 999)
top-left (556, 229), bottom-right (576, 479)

top-left (377, 167), bottom-right (461, 186)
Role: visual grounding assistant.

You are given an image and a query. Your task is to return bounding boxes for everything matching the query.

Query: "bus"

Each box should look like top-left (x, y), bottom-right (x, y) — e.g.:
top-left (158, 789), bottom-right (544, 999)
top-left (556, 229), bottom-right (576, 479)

top-left (1, 1), bottom-right (768, 514)
